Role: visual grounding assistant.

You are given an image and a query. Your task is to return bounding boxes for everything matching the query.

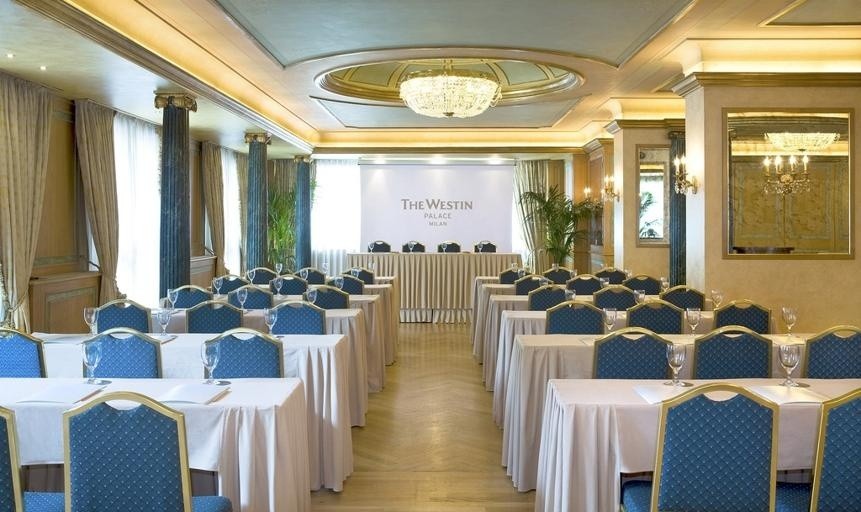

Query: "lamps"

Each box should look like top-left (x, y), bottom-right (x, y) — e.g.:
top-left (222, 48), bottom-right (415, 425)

top-left (763, 132), bottom-right (836, 153)
top-left (603, 181), bottom-right (620, 202)
top-left (584, 191), bottom-right (603, 209)
top-left (671, 157), bottom-right (698, 195)
top-left (398, 59), bottom-right (503, 119)
top-left (760, 154), bottom-right (811, 195)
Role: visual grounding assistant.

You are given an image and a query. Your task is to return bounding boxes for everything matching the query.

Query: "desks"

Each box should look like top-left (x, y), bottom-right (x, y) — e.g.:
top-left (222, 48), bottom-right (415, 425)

top-left (0, 376), bottom-right (310, 511)
top-left (471, 276), bottom-right (500, 345)
top-left (151, 309), bottom-right (370, 428)
top-left (160, 294), bottom-right (385, 394)
top-left (501, 333), bottom-right (820, 494)
top-left (534, 378), bottom-right (861, 511)
top-left (474, 284), bottom-right (621, 363)
top-left (241, 276), bottom-right (398, 359)
top-left (28, 332), bottom-right (355, 493)
top-left (346, 252), bottom-right (524, 324)
top-left (480, 294), bottom-right (714, 392)
top-left (492, 310), bottom-right (776, 430)
top-left (208, 284), bottom-right (397, 365)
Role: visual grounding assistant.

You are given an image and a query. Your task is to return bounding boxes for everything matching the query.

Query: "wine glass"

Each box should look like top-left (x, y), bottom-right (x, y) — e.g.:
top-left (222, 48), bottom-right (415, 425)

top-left (665, 343), bottom-right (688, 387)
top-left (83, 341), bottom-right (103, 384)
top-left (510, 261), bottom-right (801, 338)
top-left (778, 343), bottom-right (803, 388)
top-left (368, 241), bottom-right (375, 253)
top-left (82, 262), bottom-right (376, 340)
top-left (477, 244), bottom-right (484, 253)
top-left (441, 242), bottom-right (448, 253)
top-left (201, 341), bottom-right (221, 386)
top-left (407, 240), bottom-right (415, 253)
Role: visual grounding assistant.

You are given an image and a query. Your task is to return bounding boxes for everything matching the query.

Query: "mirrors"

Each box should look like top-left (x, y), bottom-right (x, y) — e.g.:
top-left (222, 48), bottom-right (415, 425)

top-left (721, 106), bottom-right (857, 261)
top-left (635, 143), bottom-right (670, 249)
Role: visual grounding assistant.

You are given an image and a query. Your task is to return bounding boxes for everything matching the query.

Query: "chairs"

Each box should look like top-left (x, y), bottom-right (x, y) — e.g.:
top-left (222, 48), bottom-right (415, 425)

top-left (472, 261), bottom-right (861, 512)
top-left (474, 240), bottom-right (496, 252)
top-left (437, 241), bottom-right (462, 252)
top-left (0, 270), bottom-right (399, 511)
top-left (401, 240), bottom-right (425, 252)
top-left (368, 240), bottom-right (391, 253)
